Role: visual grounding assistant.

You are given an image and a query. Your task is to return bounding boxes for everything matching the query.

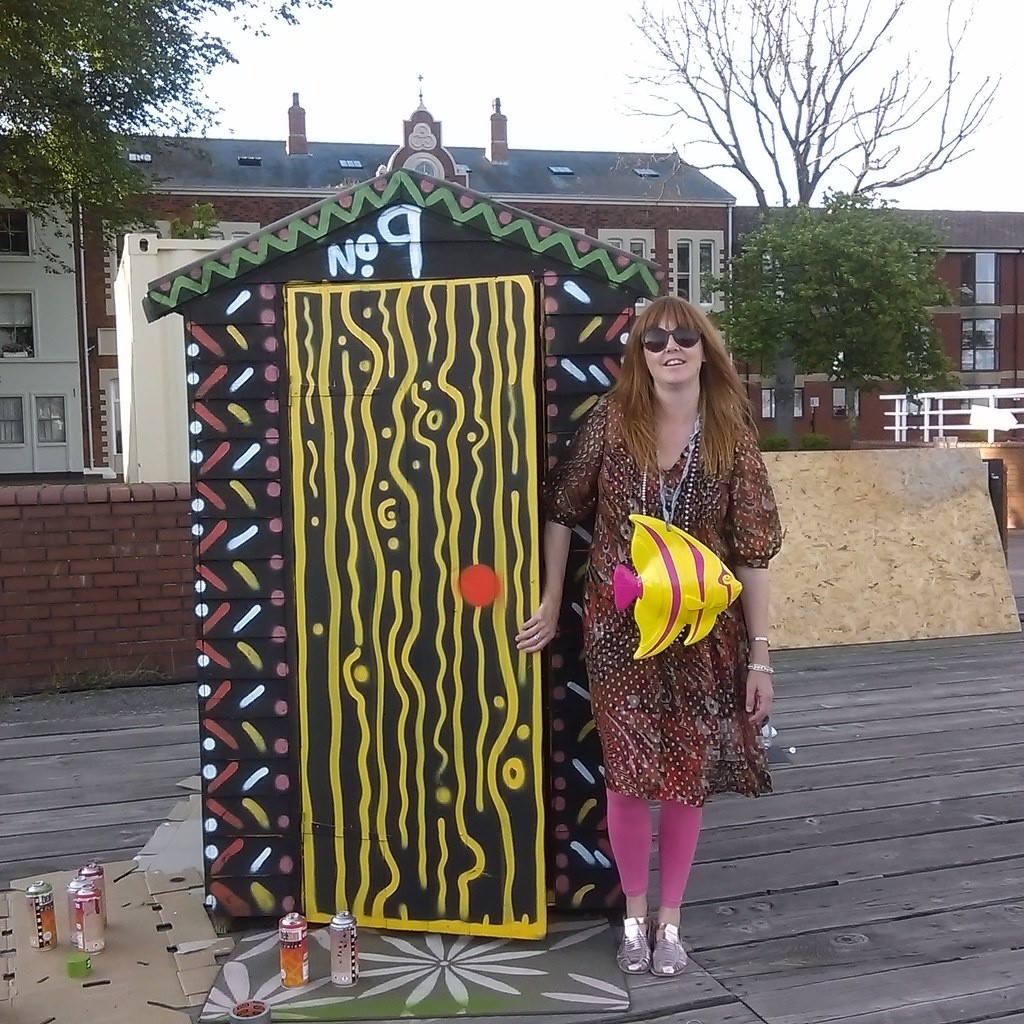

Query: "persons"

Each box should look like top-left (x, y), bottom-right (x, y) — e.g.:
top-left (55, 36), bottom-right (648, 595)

top-left (514, 296), bottom-right (783, 976)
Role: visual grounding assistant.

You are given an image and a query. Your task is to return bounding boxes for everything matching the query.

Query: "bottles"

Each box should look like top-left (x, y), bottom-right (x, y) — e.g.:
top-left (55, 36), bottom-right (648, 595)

top-left (67, 876), bottom-right (95, 944)
top-left (74, 883), bottom-right (104, 954)
top-left (279, 911), bottom-right (309, 989)
top-left (329, 910), bottom-right (358, 989)
top-left (26, 880), bottom-right (56, 952)
top-left (78, 862), bottom-right (106, 927)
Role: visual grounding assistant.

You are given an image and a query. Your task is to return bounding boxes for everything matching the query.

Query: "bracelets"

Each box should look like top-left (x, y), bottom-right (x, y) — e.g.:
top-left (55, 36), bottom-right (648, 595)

top-left (750, 637), bottom-right (771, 647)
top-left (748, 663), bottom-right (774, 675)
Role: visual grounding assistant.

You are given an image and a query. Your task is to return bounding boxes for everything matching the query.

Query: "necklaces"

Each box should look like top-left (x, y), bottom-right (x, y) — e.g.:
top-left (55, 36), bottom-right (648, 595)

top-left (641, 432), bottom-right (700, 533)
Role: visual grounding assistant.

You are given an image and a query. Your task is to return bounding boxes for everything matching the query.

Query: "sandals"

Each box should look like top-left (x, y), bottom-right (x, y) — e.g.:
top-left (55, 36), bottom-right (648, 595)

top-left (616, 904), bottom-right (652, 974)
top-left (652, 922), bottom-right (688, 976)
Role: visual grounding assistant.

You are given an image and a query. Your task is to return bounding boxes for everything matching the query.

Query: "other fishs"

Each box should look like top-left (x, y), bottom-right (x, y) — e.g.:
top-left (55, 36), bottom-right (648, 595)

top-left (610, 514), bottom-right (746, 661)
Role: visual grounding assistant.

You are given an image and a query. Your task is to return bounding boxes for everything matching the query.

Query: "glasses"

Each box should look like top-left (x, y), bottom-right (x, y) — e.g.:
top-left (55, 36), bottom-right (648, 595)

top-left (639, 323), bottom-right (702, 352)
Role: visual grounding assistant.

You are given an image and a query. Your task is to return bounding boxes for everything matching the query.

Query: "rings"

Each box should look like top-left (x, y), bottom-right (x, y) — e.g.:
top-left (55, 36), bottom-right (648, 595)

top-left (534, 635), bottom-right (540, 642)
